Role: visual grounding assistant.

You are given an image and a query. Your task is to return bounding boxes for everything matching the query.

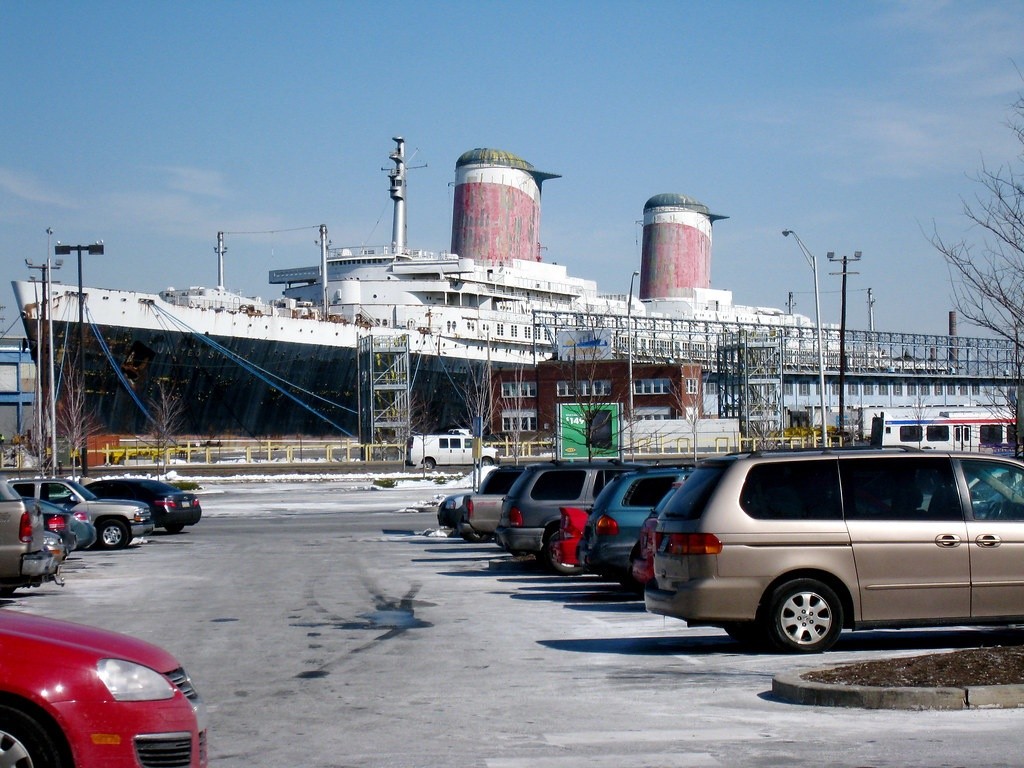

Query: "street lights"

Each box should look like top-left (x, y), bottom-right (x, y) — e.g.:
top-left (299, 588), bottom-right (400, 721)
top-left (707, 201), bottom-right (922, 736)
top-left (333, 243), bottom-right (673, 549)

top-left (626, 270), bottom-right (641, 455)
top-left (824, 249), bottom-right (862, 435)
top-left (54, 243), bottom-right (107, 474)
top-left (782, 229), bottom-right (829, 450)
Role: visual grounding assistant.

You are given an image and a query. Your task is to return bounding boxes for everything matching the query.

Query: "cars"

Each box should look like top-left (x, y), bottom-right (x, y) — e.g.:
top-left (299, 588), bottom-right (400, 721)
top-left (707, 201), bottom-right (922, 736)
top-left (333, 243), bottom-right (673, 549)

top-left (19, 496), bottom-right (98, 562)
top-left (549, 504), bottom-right (597, 568)
top-left (0, 606), bottom-right (211, 768)
top-left (630, 478), bottom-right (687, 590)
top-left (0, 476), bottom-right (66, 597)
top-left (82, 477), bottom-right (203, 533)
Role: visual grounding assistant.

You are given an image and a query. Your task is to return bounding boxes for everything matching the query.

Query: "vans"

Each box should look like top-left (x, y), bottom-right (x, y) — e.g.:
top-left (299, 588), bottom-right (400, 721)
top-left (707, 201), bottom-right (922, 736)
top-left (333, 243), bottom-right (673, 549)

top-left (405, 428), bottom-right (500, 467)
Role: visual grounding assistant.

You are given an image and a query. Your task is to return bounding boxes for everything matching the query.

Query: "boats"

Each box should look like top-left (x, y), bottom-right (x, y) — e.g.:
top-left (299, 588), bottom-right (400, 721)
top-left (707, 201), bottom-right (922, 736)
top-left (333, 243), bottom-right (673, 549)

top-left (13, 136), bottom-right (841, 438)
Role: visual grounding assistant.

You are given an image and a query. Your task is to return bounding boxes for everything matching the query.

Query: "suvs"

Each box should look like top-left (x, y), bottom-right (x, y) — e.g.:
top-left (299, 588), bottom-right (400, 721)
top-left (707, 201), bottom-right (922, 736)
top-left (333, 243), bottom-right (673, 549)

top-left (6, 478), bottom-right (157, 552)
top-left (642, 445), bottom-right (1024, 654)
top-left (436, 465), bottom-right (527, 543)
top-left (493, 456), bottom-right (631, 568)
top-left (576, 459), bottom-right (695, 588)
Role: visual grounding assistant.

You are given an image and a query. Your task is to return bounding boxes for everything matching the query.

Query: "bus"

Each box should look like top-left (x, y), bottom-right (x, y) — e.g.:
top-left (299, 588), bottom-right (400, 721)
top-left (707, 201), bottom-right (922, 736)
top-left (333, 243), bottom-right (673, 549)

top-left (872, 408), bottom-right (1018, 458)
